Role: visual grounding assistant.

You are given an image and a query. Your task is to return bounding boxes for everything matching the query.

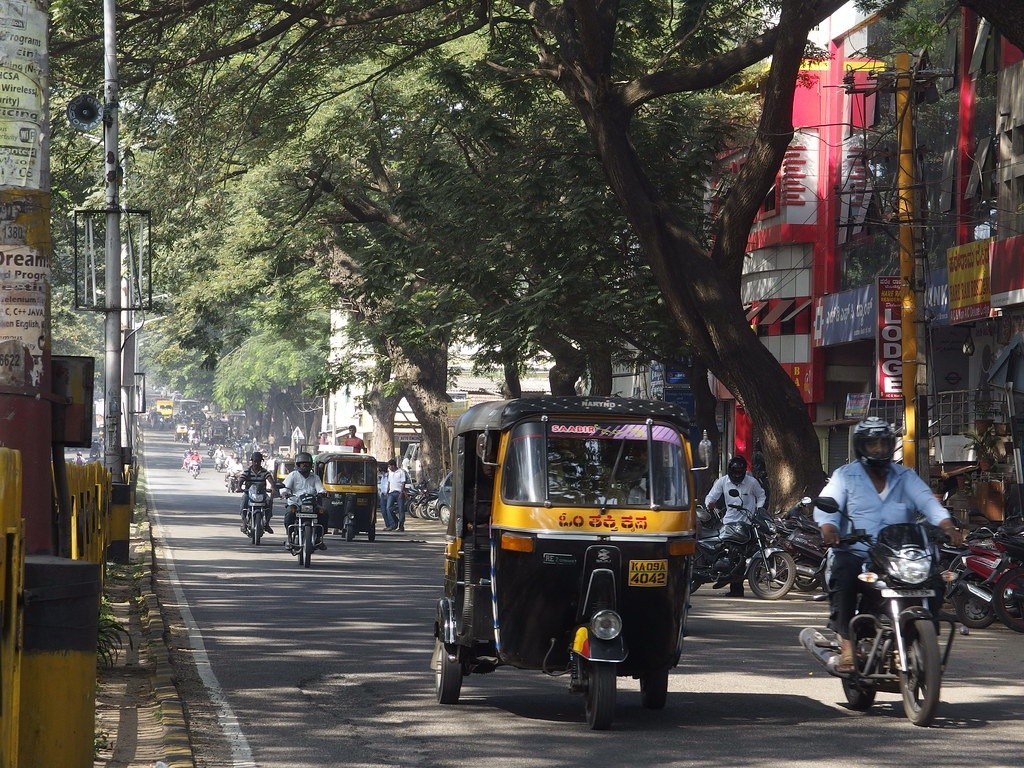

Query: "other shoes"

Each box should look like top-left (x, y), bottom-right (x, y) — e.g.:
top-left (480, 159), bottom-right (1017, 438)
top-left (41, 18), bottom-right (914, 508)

top-left (713, 581), bottom-right (728, 589)
top-left (241, 525), bottom-right (246, 532)
top-left (399, 526), bottom-right (404, 531)
top-left (316, 538), bottom-right (327, 550)
top-left (383, 525), bottom-right (394, 531)
top-left (285, 541), bottom-right (293, 550)
top-left (265, 525), bottom-right (273, 533)
top-left (727, 591), bottom-right (744, 597)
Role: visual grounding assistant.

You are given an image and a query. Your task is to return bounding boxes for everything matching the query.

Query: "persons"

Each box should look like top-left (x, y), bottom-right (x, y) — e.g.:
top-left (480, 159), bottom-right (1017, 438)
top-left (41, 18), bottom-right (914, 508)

top-left (158, 415), bottom-right (181, 429)
top-left (236, 452), bottom-right (277, 533)
top-left (188, 427), bottom-right (201, 447)
top-left (279, 452), bottom-right (328, 550)
top-left (345, 425), bottom-right (365, 453)
top-left (813, 416), bottom-right (962, 672)
top-left (705, 456), bottom-right (766, 597)
top-left (206, 415), bottom-right (290, 488)
top-left (379, 459), bottom-right (406, 531)
top-left (334, 462), bottom-right (358, 484)
top-left (465, 450), bottom-right (497, 526)
top-left (547, 439), bottom-right (625, 505)
top-left (183, 448), bottom-right (202, 473)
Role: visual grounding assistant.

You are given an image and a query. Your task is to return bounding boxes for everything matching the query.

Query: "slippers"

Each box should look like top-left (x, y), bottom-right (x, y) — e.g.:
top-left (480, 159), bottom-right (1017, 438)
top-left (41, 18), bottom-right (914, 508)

top-left (837, 656), bottom-right (856, 673)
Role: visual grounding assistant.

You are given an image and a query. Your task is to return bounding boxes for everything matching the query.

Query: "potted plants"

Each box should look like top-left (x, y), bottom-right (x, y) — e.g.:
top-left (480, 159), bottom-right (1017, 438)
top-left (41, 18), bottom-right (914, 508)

top-left (972, 399), bottom-right (994, 437)
top-left (957, 427), bottom-right (1001, 472)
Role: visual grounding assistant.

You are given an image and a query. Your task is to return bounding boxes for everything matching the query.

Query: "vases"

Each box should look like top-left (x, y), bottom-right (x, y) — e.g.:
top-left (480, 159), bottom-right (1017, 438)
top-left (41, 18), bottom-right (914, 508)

top-left (994, 421), bottom-right (1009, 436)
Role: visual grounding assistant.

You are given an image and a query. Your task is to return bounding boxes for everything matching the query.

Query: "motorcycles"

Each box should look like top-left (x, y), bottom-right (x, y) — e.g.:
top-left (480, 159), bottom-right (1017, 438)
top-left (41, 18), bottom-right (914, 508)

top-left (274, 457), bottom-right (296, 489)
top-left (798, 497), bottom-right (964, 728)
top-left (275, 482), bottom-right (332, 568)
top-left (429, 394), bottom-right (713, 733)
top-left (146, 411), bottom-right (239, 493)
top-left (687, 489), bottom-right (1023, 636)
top-left (236, 489), bottom-right (278, 546)
top-left (313, 451), bottom-right (439, 543)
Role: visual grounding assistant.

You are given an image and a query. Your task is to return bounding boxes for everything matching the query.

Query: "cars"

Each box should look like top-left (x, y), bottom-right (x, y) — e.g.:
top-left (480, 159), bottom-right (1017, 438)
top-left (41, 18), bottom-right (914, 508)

top-left (433, 471), bottom-right (453, 526)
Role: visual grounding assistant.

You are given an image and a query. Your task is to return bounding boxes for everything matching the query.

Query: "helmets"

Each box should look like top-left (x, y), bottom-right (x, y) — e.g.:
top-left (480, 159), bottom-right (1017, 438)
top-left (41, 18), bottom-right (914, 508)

top-left (853, 416), bottom-right (895, 471)
top-left (294, 452), bottom-right (313, 473)
top-left (251, 452), bottom-right (262, 460)
top-left (727, 455), bottom-right (747, 485)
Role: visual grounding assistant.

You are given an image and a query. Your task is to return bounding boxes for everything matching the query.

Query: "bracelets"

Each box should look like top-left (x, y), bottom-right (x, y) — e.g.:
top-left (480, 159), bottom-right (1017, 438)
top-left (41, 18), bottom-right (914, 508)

top-left (944, 525), bottom-right (956, 530)
top-left (401, 492), bottom-right (404, 494)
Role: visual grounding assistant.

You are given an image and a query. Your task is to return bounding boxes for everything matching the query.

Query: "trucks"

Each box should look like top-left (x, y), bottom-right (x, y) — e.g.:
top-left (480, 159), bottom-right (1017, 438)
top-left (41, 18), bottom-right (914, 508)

top-left (156, 400), bottom-right (174, 420)
top-left (399, 442), bottom-right (421, 483)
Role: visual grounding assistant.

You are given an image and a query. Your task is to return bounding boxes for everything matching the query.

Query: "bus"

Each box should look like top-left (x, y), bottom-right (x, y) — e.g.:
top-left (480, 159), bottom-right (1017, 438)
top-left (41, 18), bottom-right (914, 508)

top-left (178, 399), bottom-right (200, 415)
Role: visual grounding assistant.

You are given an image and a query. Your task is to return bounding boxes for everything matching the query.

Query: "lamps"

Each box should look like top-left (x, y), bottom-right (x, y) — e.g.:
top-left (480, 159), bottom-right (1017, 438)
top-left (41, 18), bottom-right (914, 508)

top-left (953, 322), bottom-right (976, 356)
